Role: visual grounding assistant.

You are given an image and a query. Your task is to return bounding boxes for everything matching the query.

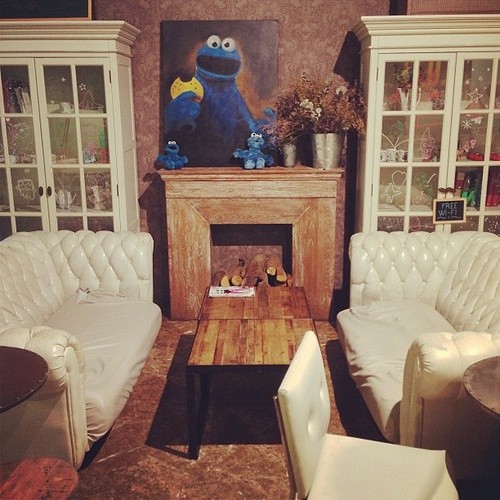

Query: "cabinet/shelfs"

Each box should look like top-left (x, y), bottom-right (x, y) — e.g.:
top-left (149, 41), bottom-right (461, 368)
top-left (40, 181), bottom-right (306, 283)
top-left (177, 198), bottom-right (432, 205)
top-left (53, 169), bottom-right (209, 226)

top-left (0, 18), bottom-right (142, 230)
top-left (353, 14), bottom-right (500, 235)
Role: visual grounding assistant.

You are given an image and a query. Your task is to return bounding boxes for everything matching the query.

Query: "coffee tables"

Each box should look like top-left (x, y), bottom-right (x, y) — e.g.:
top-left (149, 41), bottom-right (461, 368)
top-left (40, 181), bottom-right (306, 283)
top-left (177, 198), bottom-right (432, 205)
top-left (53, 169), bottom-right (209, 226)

top-left (186, 284), bottom-right (318, 458)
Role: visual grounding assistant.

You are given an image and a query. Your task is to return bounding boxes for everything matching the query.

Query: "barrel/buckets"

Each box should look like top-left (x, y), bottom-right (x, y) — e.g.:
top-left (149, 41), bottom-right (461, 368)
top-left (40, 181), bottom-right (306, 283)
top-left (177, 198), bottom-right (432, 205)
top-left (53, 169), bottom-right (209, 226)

top-left (311, 133), bottom-right (343, 168)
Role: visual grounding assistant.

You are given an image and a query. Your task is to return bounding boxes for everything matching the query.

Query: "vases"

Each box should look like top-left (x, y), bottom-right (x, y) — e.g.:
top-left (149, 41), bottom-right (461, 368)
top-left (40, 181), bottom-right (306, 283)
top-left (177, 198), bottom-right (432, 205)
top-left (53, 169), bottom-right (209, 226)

top-left (312, 132), bottom-right (344, 169)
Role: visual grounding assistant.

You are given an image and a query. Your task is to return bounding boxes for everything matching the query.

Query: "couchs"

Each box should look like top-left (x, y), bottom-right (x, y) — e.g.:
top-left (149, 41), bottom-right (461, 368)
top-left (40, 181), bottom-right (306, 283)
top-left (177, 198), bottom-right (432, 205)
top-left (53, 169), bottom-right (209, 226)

top-left (0, 229), bottom-right (162, 471)
top-left (336, 230), bottom-right (500, 481)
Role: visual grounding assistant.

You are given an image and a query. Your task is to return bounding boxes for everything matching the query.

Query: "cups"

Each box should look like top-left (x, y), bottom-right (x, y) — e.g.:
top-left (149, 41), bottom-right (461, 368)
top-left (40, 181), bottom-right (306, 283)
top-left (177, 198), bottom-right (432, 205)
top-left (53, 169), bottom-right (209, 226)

top-left (386, 149), bottom-right (395, 162)
top-left (381, 150), bottom-right (387, 161)
top-left (395, 150), bottom-right (407, 162)
top-left (52, 154), bottom-right (56, 163)
top-left (10, 155), bottom-right (19, 165)
top-left (60, 102), bottom-right (74, 113)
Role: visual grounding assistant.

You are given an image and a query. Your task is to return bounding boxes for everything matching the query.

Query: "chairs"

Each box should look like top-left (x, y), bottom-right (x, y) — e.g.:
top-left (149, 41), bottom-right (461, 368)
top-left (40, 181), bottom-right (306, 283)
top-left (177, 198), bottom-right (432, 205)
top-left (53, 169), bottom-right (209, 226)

top-left (274, 331), bottom-right (462, 500)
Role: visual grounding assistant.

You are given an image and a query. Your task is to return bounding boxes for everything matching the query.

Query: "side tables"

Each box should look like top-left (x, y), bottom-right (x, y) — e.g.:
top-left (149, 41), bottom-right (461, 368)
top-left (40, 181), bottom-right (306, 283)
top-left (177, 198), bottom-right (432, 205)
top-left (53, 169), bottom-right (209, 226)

top-left (0, 344), bottom-right (80, 500)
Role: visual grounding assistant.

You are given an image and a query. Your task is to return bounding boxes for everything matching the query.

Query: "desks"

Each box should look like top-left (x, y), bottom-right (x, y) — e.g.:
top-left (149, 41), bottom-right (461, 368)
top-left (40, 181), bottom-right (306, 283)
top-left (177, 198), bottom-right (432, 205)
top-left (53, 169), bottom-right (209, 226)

top-left (160, 163), bottom-right (346, 318)
top-left (463, 356), bottom-right (500, 421)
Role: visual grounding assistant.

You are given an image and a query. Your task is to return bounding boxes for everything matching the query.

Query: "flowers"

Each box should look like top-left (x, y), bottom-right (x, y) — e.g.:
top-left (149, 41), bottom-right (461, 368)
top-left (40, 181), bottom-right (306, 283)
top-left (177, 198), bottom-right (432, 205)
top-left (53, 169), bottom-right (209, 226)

top-left (270, 70), bottom-right (367, 140)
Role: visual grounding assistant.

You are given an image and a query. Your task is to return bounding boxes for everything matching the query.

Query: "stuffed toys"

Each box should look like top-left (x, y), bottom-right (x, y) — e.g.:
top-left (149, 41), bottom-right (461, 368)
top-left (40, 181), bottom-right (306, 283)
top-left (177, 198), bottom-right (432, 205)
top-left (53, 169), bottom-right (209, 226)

top-left (157, 141), bottom-right (188, 169)
top-left (234, 133), bottom-right (273, 169)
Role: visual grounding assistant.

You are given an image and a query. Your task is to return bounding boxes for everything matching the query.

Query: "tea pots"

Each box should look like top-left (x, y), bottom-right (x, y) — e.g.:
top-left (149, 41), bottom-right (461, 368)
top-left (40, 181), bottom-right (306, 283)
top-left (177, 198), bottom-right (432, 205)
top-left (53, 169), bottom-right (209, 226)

top-left (88, 185), bottom-right (110, 210)
top-left (54, 189), bottom-right (77, 209)
